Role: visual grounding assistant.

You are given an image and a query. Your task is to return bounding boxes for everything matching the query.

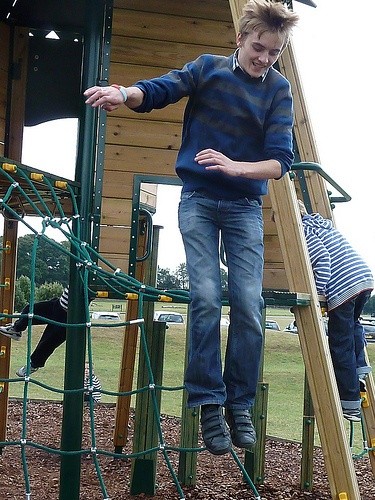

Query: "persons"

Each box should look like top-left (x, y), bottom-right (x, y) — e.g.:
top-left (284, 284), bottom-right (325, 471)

top-left (0, 284), bottom-right (69, 377)
top-left (82, 0), bottom-right (299, 455)
top-left (84, 361), bottom-right (102, 403)
top-left (271, 197), bottom-right (374, 423)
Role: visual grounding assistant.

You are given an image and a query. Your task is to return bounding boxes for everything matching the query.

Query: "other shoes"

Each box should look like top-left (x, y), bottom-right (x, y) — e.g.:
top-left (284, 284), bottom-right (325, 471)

top-left (359, 376), bottom-right (367, 393)
top-left (1, 324), bottom-right (23, 341)
top-left (15, 363), bottom-right (39, 378)
top-left (343, 412), bottom-right (361, 422)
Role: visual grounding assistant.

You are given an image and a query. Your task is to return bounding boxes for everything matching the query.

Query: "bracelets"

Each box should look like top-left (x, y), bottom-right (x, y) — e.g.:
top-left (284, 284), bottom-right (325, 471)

top-left (111, 84), bottom-right (128, 103)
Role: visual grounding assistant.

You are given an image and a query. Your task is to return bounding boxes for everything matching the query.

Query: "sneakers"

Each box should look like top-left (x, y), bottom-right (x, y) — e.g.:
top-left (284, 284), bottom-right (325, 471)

top-left (225, 407), bottom-right (257, 448)
top-left (200, 405), bottom-right (232, 456)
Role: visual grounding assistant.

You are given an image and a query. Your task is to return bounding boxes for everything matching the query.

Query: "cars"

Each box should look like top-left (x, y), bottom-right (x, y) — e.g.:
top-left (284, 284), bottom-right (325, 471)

top-left (283, 320), bottom-right (298, 334)
top-left (154, 311), bottom-right (185, 324)
top-left (89, 310), bottom-right (121, 320)
top-left (358, 314), bottom-right (375, 342)
top-left (265, 320), bottom-right (281, 331)
